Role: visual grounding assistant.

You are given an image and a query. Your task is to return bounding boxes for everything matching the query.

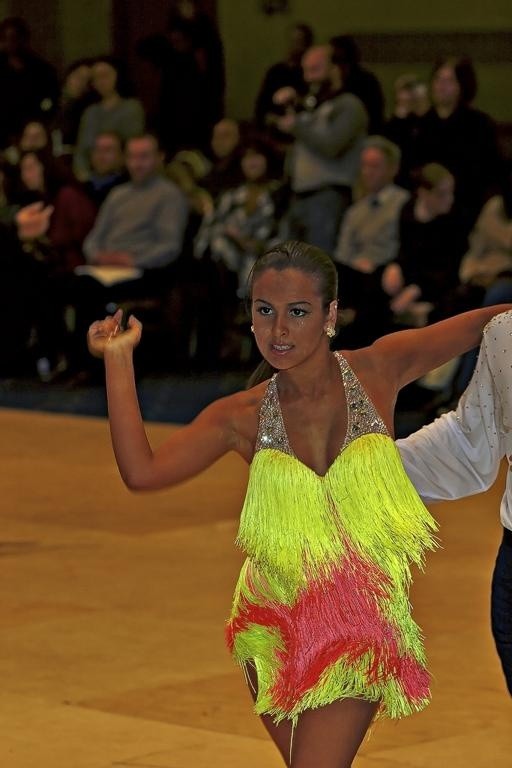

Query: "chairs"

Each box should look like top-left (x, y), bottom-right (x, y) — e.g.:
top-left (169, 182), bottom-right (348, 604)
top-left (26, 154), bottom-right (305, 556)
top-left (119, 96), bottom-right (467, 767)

top-left (73, 185), bottom-right (214, 377)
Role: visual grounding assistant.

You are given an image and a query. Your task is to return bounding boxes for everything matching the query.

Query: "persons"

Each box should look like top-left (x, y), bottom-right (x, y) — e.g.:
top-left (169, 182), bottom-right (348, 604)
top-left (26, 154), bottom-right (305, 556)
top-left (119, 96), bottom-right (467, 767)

top-left (1, 1), bottom-right (512, 437)
top-left (395, 307), bottom-right (512, 696)
top-left (89, 241), bottom-right (512, 768)
